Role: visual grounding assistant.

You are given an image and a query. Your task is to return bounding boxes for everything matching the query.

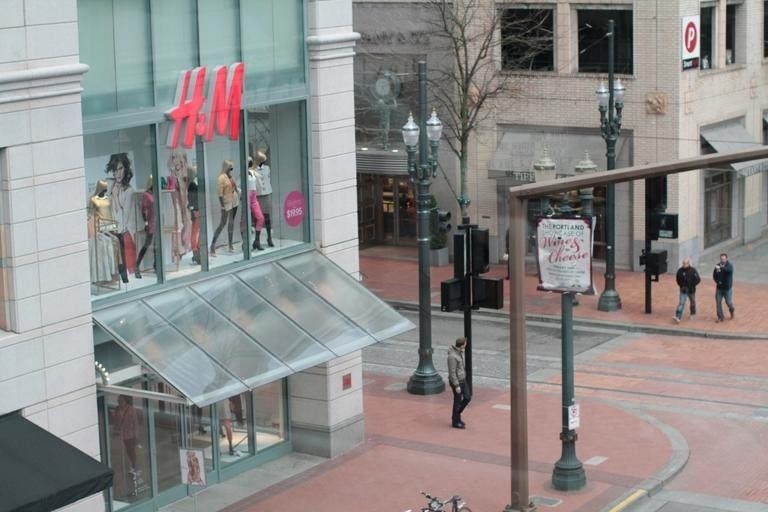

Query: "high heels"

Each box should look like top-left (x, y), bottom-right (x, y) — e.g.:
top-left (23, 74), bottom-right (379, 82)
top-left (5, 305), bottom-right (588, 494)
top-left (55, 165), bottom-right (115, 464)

top-left (253, 240), bottom-right (264, 250)
top-left (267, 238), bottom-right (274, 246)
top-left (192, 256), bottom-right (201, 265)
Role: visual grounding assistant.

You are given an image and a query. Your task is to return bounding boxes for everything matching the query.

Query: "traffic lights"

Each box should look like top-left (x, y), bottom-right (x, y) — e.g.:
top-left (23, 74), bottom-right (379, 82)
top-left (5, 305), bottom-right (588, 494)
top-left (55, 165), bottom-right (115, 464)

top-left (440, 279), bottom-right (460, 312)
top-left (650, 248), bottom-right (667, 274)
top-left (430, 208), bottom-right (454, 251)
top-left (652, 173), bottom-right (667, 213)
top-left (469, 226), bottom-right (489, 275)
top-left (656, 213), bottom-right (677, 239)
top-left (472, 275), bottom-right (502, 309)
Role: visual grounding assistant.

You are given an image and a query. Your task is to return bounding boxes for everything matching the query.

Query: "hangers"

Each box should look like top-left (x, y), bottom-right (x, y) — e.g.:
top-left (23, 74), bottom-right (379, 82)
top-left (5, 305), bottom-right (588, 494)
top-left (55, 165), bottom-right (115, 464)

top-left (93, 222), bottom-right (121, 239)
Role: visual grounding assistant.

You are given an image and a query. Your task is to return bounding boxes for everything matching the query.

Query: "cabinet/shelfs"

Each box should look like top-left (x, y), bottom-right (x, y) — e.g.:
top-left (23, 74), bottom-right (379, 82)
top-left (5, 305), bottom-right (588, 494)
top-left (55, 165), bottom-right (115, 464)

top-left (132, 187), bottom-right (181, 276)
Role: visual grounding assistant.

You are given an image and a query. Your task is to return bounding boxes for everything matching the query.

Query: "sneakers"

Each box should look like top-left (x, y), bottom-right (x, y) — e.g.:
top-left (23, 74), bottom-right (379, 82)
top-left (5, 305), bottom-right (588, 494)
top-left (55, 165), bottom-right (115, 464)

top-left (450, 415), bottom-right (466, 428)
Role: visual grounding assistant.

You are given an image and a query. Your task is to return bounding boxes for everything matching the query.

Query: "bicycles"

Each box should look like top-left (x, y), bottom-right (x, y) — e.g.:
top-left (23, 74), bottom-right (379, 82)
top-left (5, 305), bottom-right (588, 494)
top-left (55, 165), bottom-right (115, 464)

top-left (418, 491), bottom-right (472, 512)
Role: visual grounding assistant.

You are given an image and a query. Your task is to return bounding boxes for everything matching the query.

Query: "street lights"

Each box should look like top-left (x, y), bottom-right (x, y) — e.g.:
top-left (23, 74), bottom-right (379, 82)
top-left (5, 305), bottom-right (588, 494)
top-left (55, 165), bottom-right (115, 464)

top-left (400, 62), bottom-right (447, 397)
top-left (533, 146), bottom-right (597, 490)
top-left (595, 19), bottom-right (626, 314)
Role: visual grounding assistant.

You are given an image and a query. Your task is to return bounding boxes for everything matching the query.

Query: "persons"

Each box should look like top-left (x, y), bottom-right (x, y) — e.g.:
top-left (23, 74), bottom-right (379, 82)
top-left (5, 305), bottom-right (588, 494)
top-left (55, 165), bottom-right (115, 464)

top-left (187, 166), bottom-right (201, 265)
top-left (713, 253), bottom-right (735, 323)
top-left (114, 393), bottom-right (139, 477)
top-left (210, 159), bottom-right (242, 257)
top-left (448, 337), bottom-right (471, 430)
top-left (135, 174), bottom-right (157, 278)
top-left (105, 152), bottom-right (138, 274)
top-left (239, 156), bottom-right (265, 250)
top-left (219, 398), bottom-right (235, 455)
top-left (399, 189), bottom-right (415, 237)
top-left (671, 258), bottom-right (701, 323)
top-left (167, 140), bottom-right (191, 262)
top-left (86, 179), bottom-right (129, 283)
top-left (253, 150), bottom-right (275, 246)
top-left (190, 404), bottom-right (207, 436)
